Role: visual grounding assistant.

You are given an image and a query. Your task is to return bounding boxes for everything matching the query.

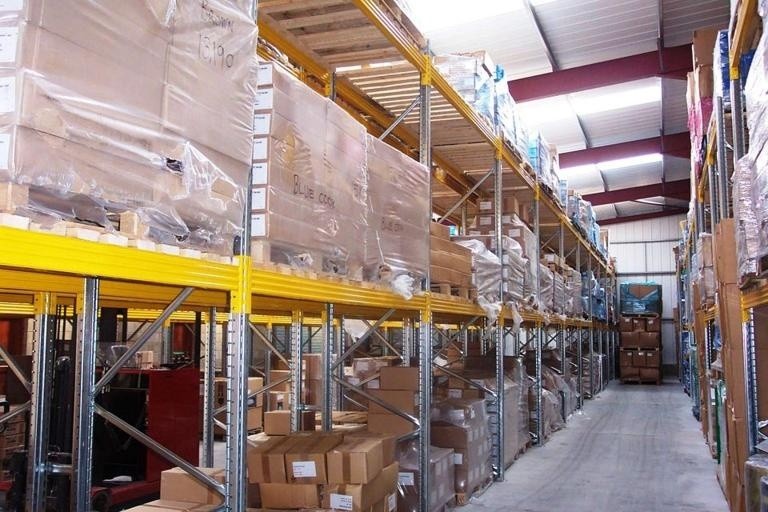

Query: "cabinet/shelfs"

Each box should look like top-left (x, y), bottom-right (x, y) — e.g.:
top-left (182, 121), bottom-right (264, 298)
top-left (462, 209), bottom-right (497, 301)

top-left (3, 365), bottom-right (202, 509)
top-left (675, 0), bottom-right (768, 511)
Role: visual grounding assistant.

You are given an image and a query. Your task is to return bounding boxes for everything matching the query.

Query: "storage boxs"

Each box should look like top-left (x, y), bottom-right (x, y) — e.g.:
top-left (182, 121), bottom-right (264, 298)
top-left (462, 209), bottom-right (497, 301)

top-left (0, 0), bottom-right (259, 260)
top-left (619, 284), bottom-right (663, 386)
top-left (429, 189), bottom-right (618, 322)
top-left (245, 60), bottom-right (430, 293)
top-left (432, 48), bottom-right (562, 191)
top-left (124, 342), bottom-right (605, 511)
